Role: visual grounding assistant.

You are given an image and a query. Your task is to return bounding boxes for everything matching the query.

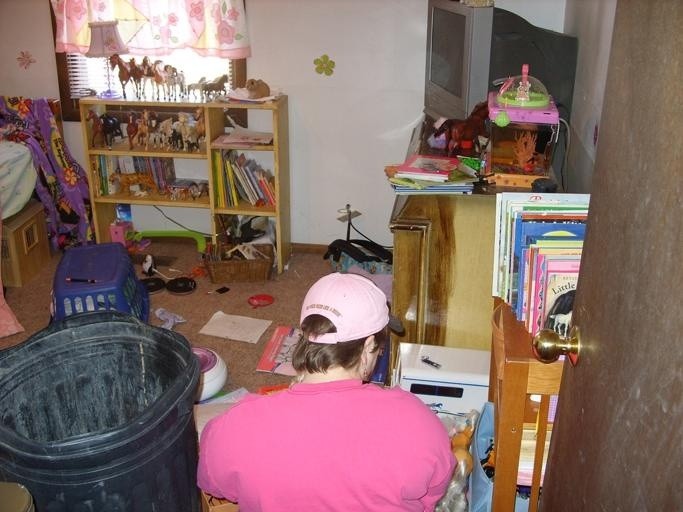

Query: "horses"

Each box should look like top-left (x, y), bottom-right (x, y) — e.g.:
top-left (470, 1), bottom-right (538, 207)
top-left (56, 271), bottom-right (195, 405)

top-left (84, 109), bottom-right (203, 152)
top-left (434, 100), bottom-right (491, 156)
top-left (107, 52), bottom-right (230, 101)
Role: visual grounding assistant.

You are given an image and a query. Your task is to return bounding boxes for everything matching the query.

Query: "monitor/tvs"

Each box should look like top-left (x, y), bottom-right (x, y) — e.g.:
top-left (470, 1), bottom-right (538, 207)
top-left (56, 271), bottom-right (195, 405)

top-left (422, 0), bottom-right (578, 126)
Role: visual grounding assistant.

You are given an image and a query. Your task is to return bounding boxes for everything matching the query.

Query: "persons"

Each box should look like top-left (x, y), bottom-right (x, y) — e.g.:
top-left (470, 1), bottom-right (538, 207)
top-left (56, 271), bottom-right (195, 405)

top-left (197, 268), bottom-right (458, 512)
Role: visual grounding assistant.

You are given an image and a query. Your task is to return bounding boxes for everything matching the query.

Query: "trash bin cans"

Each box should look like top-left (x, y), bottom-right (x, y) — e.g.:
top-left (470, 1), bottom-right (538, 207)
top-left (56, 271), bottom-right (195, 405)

top-left (0, 309), bottom-right (200, 512)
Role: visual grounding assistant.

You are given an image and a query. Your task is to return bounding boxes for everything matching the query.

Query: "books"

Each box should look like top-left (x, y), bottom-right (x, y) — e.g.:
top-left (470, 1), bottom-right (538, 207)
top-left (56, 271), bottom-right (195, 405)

top-left (208, 128), bottom-right (276, 209)
top-left (492, 190), bottom-right (593, 339)
top-left (384, 154), bottom-right (480, 196)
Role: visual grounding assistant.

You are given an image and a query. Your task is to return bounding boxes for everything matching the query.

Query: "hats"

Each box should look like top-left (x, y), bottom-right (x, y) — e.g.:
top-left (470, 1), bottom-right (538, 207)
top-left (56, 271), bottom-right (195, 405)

top-left (299, 271), bottom-right (405, 345)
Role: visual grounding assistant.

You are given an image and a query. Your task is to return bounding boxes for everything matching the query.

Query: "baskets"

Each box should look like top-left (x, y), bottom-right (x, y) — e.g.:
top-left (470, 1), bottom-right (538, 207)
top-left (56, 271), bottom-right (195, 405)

top-left (206, 242), bottom-right (274, 284)
top-left (48, 242), bottom-right (149, 327)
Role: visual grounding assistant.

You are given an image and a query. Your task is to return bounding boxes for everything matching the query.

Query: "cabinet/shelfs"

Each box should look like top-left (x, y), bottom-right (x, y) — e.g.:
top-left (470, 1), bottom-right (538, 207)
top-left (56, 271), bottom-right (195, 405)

top-left (387, 118), bottom-right (565, 511)
top-left (77, 95), bottom-right (290, 274)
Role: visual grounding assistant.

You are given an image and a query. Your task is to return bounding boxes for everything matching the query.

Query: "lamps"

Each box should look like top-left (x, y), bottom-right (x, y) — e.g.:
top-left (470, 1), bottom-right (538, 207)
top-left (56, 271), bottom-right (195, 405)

top-left (84, 20), bottom-right (129, 100)
